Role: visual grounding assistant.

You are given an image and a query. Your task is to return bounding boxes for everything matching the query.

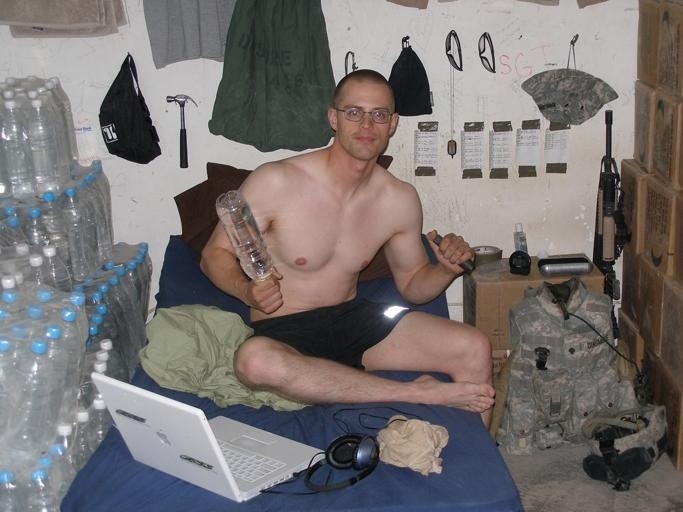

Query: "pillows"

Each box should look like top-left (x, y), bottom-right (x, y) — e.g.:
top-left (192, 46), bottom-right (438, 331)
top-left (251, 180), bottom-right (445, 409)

top-left (174, 162), bottom-right (256, 250)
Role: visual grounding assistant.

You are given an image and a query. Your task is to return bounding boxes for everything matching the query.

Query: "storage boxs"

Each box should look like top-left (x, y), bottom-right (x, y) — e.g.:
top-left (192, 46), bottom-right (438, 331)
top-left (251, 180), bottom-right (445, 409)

top-left (617, 1), bottom-right (683, 469)
top-left (461, 253), bottom-right (608, 386)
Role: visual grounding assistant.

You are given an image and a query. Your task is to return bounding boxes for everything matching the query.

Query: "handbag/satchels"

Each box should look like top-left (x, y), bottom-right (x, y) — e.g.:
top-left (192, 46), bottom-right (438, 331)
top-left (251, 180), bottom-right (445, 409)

top-left (99, 56), bottom-right (161, 164)
top-left (387, 48), bottom-right (432, 116)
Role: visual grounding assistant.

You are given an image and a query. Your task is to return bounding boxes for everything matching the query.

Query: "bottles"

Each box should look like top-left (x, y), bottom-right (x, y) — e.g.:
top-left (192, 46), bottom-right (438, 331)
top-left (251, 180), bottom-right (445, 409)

top-left (0, 74), bottom-right (152, 512)
top-left (512, 222), bottom-right (528, 254)
top-left (215, 189), bottom-right (273, 279)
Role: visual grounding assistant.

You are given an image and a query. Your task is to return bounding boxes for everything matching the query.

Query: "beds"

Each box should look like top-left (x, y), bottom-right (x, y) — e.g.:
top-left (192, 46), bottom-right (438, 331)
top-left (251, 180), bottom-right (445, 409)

top-left (56, 235), bottom-right (527, 512)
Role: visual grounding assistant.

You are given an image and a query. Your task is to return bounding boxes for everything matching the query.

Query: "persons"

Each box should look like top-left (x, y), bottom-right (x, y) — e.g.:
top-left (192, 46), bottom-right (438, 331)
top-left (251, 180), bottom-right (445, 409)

top-left (199, 70), bottom-right (497, 429)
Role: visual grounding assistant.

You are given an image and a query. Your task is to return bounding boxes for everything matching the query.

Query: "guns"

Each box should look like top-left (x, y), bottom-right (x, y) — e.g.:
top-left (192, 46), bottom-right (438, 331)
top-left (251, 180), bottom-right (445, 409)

top-left (598, 110), bottom-right (631, 341)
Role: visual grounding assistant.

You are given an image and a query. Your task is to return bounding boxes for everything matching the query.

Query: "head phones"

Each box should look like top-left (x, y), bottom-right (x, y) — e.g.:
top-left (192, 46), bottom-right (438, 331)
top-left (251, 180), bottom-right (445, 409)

top-left (304, 435), bottom-right (378, 491)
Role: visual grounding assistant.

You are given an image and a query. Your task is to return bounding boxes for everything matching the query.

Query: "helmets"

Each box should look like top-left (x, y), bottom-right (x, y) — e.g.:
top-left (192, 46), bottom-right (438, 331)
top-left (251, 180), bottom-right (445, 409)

top-left (579, 405), bottom-right (668, 489)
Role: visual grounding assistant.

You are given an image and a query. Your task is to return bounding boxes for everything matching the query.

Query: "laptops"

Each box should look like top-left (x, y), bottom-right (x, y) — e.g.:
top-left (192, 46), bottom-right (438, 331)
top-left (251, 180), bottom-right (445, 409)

top-left (91, 372), bottom-right (327, 503)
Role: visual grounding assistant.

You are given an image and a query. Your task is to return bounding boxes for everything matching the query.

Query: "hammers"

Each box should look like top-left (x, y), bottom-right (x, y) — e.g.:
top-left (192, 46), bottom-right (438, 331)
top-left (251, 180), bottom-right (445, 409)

top-left (166, 95), bottom-right (198, 168)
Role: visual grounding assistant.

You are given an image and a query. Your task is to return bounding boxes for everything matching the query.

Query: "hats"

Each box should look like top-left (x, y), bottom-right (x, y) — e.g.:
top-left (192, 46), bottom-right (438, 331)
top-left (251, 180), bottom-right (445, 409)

top-left (522, 69), bottom-right (619, 125)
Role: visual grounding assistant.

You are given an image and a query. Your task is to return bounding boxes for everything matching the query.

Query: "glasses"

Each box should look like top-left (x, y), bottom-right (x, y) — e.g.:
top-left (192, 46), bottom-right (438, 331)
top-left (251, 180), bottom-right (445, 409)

top-left (479, 32), bottom-right (495, 72)
top-left (332, 105), bottom-right (393, 125)
top-left (446, 30), bottom-right (462, 72)
